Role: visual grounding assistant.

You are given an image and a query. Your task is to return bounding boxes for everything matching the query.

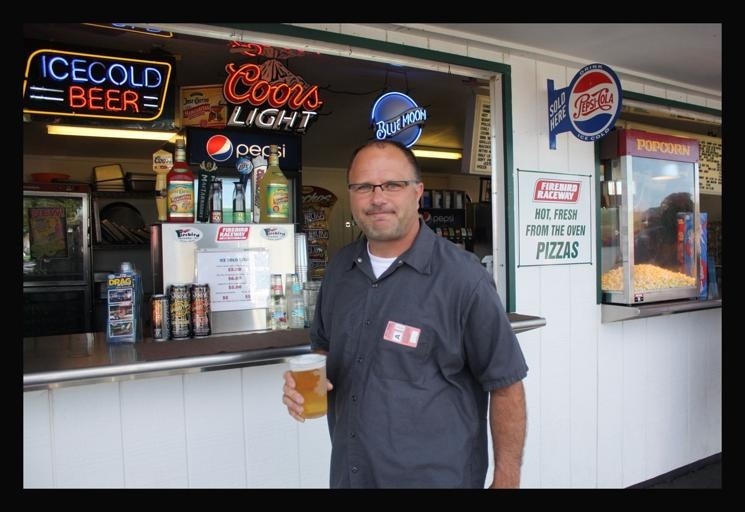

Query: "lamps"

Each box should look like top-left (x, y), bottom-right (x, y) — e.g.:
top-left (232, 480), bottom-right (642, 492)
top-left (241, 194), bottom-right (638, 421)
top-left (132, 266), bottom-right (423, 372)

top-left (410, 145), bottom-right (466, 162)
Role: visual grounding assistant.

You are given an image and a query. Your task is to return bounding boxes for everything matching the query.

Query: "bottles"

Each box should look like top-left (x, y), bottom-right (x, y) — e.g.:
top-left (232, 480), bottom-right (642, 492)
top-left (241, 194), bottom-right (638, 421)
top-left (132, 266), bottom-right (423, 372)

top-left (164, 138), bottom-right (194, 225)
top-left (286, 273), bottom-right (304, 330)
top-left (231, 181), bottom-right (247, 224)
top-left (208, 181), bottom-right (222, 223)
top-left (258, 144), bottom-right (290, 225)
top-left (267, 274), bottom-right (287, 332)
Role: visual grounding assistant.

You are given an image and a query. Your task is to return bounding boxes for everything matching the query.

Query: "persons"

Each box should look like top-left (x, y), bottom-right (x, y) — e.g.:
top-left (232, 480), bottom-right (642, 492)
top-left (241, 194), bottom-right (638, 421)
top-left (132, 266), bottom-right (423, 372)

top-left (282, 140), bottom-right (529, 489)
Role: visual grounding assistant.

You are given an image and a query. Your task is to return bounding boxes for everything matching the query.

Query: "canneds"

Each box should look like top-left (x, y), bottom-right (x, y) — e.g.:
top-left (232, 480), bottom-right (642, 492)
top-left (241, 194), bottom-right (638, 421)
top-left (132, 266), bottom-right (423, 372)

top-left (170, 284), bottom-right (192, 341)
top-left (150, 294), bottom-right (170, 343)
top-left (192, 283), bottom-right (211, 336)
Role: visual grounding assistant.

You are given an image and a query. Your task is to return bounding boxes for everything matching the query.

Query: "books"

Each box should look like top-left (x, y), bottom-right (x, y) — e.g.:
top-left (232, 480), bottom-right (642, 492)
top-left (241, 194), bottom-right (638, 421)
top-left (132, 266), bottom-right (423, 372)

top-left (101, 219), bottom-right (150, 244)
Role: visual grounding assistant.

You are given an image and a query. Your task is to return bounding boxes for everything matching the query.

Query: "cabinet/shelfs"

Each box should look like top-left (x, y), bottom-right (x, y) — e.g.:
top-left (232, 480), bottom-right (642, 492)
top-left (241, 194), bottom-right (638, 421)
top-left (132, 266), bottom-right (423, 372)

top-left (23, 190), bottom-right (95, 336)
top-left (92, 192), bottom-right (156, 251)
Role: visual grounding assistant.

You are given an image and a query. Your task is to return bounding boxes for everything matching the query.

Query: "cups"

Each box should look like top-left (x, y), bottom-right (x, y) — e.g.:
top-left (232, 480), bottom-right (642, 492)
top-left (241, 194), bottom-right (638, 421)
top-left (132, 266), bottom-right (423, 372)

top-left (293, 231), bottom-right (323, 329)
top-left (283, 354), bottom-right (328, 420)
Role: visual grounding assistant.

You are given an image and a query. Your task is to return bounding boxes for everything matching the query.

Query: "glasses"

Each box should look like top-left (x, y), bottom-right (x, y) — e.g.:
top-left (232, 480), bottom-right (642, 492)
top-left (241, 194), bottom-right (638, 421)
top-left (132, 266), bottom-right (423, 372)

top-left (347, 180), bottom-right (419, 194)
